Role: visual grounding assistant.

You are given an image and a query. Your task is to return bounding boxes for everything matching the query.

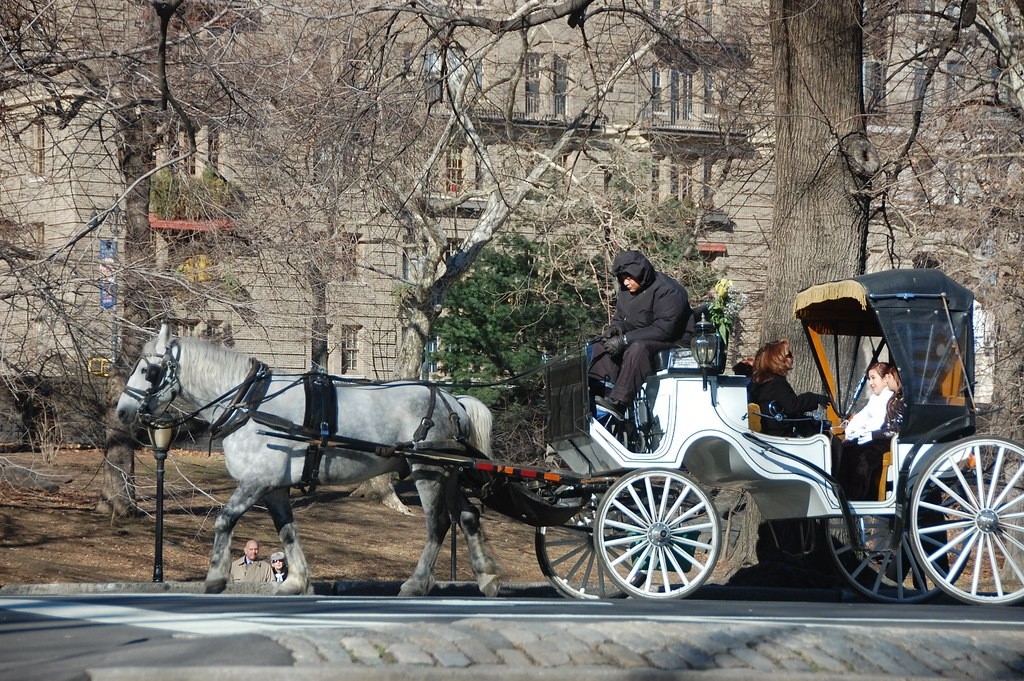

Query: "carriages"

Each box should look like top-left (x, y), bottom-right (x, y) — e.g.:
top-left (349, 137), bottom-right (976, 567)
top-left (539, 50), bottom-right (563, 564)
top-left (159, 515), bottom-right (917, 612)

top-left (111, 266), bottom-right (1024, 607)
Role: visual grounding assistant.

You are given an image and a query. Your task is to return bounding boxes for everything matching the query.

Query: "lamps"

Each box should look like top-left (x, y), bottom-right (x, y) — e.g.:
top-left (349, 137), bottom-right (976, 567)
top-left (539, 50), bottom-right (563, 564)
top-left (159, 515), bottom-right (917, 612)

top-left (692, 311), bottom-right (723, 392)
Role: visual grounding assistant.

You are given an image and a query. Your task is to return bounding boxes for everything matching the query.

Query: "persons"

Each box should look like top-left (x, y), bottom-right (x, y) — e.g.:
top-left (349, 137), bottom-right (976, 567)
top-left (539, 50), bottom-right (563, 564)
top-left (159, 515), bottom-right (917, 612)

top-left (588, 251), bottom-right (698, 422)
top-left (746, 340), bottom-right (842, 478)
top-left (843, 362), bottom-right (894, 453)
top-left (842, 366), bottom-right (903, 501)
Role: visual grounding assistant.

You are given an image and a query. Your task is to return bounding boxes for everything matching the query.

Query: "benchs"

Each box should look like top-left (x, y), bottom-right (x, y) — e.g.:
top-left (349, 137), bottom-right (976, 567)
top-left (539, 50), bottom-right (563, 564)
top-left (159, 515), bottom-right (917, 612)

top-left (650, 304), bottom-right (733, 374)
top-left (832, 425), bottom-right (892, 502)
top-left (747, 401), bottom-right (828, 439)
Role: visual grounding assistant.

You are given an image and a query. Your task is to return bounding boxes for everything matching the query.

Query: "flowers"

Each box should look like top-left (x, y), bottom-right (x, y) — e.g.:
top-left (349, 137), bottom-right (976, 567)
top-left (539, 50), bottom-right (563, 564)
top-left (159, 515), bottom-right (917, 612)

top-left (702, 277), bottom-right (746, 344)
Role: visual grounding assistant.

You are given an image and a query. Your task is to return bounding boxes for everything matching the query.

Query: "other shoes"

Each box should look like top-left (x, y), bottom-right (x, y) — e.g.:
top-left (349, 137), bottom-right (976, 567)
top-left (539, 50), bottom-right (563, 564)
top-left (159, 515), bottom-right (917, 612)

top-left (595, 395), bottom-right (628, 421)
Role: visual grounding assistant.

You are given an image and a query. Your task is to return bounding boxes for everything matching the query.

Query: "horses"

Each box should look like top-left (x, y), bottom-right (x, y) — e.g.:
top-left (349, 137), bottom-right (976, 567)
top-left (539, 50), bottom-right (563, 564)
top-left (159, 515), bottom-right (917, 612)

top-left (116, 318), bottom-right (503, 600)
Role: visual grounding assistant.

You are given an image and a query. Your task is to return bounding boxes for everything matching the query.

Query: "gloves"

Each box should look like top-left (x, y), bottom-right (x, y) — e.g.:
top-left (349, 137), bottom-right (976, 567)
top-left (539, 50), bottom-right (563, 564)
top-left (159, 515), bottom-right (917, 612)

top-left (600, 327), bottom-right (617, 342)
top-left (604, 334), bottom-right (626, 358)
top-left (817, 394), bottom-right (832, 409)
top-left (857, 431), bottom-right (873, 445)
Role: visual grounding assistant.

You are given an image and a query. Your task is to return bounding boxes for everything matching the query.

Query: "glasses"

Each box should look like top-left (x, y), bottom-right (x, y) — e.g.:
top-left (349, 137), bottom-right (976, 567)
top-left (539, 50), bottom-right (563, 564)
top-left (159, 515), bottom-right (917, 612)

top-left (272, 559), bottom-right (284, 563)
top-left (785, 351), bottom-right (792, 358)
